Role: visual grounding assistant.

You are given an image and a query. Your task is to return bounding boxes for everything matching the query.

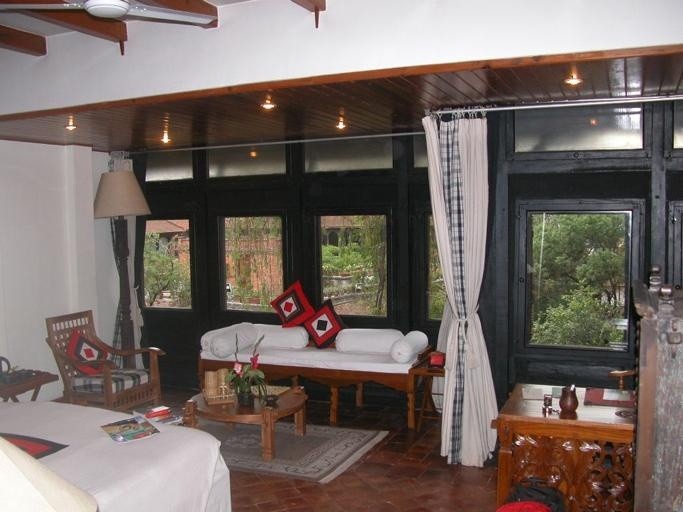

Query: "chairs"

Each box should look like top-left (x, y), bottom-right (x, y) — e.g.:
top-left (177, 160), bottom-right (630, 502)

top-left (46, 310), bottom-right (168, 411)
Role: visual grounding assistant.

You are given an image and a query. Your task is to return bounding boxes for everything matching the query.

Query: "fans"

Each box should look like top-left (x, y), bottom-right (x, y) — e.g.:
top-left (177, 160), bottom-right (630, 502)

top-left (0, 0), bottom-right (217, 25)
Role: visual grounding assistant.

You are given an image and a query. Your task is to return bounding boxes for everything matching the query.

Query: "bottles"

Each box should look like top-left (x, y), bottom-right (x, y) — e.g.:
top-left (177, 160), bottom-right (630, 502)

top-left (559, 387), bottom-right (578, 412)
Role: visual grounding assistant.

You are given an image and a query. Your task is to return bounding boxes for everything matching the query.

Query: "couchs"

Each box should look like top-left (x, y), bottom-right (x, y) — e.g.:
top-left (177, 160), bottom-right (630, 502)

top-left (200, 324), bottom-right (432, 428)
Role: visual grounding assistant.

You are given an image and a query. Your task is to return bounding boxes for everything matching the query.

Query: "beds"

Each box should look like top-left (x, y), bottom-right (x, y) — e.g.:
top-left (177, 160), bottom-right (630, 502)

top-left (0, 400), bottom-right (232, 512)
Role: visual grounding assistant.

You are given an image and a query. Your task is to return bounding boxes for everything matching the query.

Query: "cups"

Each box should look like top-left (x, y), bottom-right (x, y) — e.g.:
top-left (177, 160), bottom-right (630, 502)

top-left (217, 368), bottom-right (230, 396)
top-left (204, 371), bottom-right (217, 396)
top-left (544, 393), bottom-right (553, 409)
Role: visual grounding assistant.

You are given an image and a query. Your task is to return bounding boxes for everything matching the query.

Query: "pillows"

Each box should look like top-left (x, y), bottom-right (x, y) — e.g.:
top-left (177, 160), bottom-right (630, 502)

top-left (210, 280), bottom-right (428, 364)
top-left (65, 329), bottom-right (108, 376)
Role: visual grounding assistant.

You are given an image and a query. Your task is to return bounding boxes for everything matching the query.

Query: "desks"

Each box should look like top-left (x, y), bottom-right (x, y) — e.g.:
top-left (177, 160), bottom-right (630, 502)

top-left (410, 355), bottom-right (446, 432)
top-left (0, 370), bottom-right (59, 402)
top-left (497, 383), bottom-right (637, 512)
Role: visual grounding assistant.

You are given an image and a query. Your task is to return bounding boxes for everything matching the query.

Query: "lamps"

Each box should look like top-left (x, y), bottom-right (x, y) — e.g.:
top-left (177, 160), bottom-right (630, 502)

top-left (94, 170), bottom-right (152, 369)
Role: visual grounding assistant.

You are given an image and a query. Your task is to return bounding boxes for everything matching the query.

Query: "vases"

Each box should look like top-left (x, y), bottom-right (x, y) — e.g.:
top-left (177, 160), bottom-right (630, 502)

top-left (238, 390), bottom-right (256, 408)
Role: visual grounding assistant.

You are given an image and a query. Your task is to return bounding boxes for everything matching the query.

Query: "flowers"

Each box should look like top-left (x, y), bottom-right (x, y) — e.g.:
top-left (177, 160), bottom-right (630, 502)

top-left (225, 331), bottom-right (268, 407)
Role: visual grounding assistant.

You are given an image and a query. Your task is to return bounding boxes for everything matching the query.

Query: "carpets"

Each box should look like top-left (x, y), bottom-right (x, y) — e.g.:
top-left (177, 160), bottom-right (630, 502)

top-left (155, 405), bottom-right (390, 484)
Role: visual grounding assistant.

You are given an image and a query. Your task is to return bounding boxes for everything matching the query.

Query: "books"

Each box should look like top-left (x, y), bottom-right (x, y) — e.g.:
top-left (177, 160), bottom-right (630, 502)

top-left (100, 416), bottom-right (160, 443)
top-left (132, 402), bottom-right (182, 425)
